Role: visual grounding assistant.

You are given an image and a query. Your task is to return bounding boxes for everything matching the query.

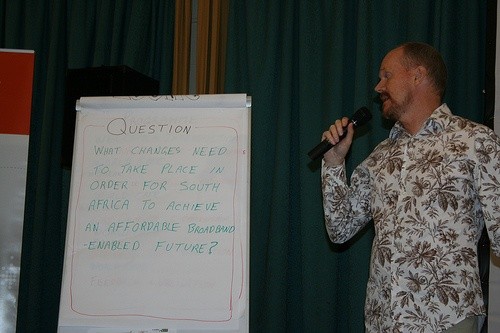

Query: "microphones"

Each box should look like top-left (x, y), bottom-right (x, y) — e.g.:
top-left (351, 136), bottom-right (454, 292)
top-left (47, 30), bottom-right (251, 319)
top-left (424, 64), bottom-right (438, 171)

top-left (306, 106), bottom-right (373, 160)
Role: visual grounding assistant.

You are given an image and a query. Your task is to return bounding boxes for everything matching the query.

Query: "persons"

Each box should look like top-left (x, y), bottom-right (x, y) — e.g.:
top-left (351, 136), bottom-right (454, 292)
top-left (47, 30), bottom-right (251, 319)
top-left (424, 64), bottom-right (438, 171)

top-left (319, 40), bottom-right (499, 333)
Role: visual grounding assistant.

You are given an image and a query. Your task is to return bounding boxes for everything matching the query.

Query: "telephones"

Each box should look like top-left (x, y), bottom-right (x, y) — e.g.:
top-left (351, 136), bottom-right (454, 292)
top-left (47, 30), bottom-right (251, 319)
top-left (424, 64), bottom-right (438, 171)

top-left (56, 94), bottom-right (254, 332)
